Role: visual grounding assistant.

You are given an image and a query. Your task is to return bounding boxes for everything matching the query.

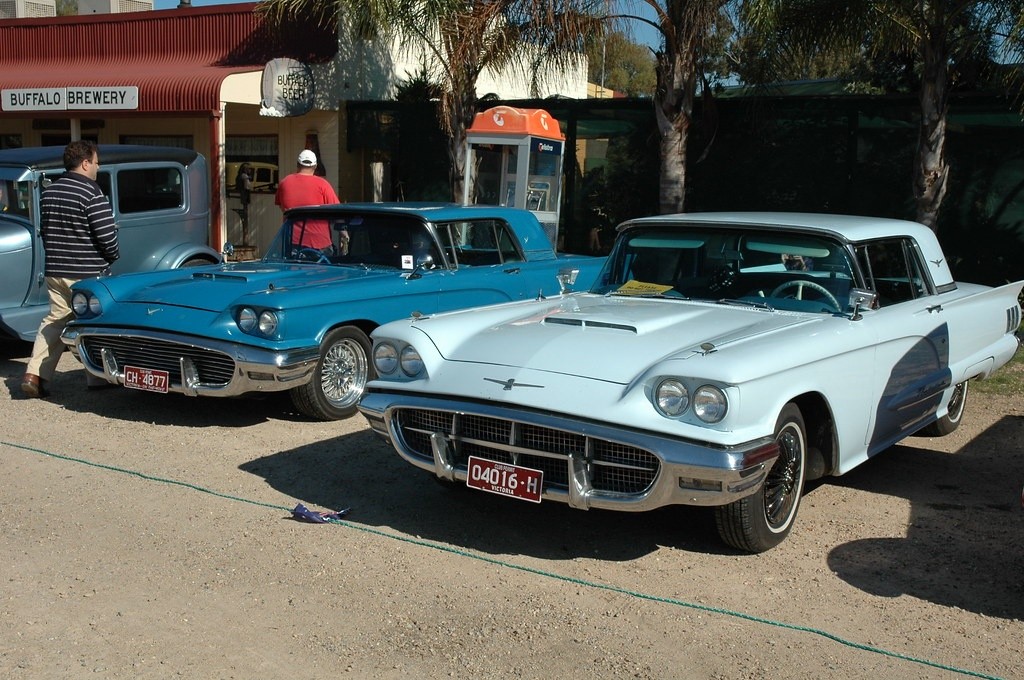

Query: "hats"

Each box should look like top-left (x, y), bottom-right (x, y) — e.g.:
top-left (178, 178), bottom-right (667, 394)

top-left (298, 149), bottom-right (317, 166)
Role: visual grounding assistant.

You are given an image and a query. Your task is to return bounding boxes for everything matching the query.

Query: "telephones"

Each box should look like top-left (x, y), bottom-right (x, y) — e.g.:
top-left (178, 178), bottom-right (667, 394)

top-left (527, 190), bottom-right (546, 210)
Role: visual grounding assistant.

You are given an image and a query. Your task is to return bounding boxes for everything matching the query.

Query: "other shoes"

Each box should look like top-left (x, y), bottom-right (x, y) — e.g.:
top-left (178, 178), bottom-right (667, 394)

top-left (88, 384), bottom-right (121, 390)
top-left (20, 373), bottom-right (51, 398)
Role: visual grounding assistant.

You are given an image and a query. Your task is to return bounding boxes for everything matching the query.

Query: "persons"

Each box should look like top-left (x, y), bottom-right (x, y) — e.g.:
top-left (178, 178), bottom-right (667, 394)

top-left (20, 139), bottom-right (120, 399)
top-left (270, 148), bottom-right (354, 261)
top-left (235, 163), bottom-right (255, 191)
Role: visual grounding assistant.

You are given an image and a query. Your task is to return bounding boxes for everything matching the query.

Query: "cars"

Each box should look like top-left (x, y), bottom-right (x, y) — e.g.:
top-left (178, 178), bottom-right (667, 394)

top-left (0, 143), bottom-right (222, 377)
top-left (357, 209), bottom-right (1024, 556)
top-left (57, 201), bottom-right (636, 421)
